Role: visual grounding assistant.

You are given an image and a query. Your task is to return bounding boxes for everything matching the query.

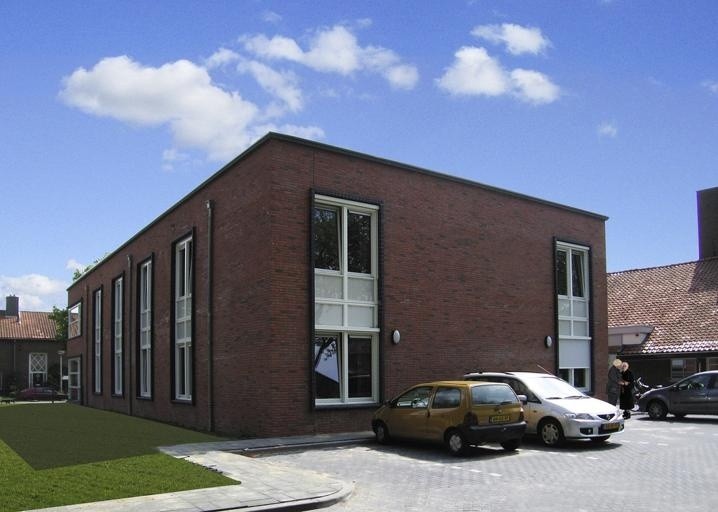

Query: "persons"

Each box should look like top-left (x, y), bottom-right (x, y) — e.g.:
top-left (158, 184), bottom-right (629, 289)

top-left (606, 358), bottom-right (630, 407)
top-left (620, 362), bottom-right (635, 420)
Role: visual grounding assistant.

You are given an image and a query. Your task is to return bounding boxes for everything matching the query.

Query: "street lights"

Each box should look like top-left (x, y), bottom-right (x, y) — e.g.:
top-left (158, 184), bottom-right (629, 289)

top-left (56, 350), bottom-right (65, 394)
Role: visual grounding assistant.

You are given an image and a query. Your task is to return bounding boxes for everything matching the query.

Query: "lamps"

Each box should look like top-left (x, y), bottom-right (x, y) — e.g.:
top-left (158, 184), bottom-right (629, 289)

top-left (392, 328), bottom-right (400, 344)
top-left (545, 336), bottom-right (552, 348)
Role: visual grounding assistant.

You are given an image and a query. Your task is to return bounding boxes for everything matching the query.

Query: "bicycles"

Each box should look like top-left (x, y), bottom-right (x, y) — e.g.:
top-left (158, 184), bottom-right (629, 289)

top-left (630, 377), bottom-right (650, 412)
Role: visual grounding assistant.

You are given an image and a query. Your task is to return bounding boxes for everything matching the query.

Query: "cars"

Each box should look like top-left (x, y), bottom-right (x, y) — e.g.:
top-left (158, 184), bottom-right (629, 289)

top-left (638, 370), bottom-right (718, 419)
top-left (17, 388), bottom-right (67, 401)
top-left (372, 381), bottom-right (524, 456)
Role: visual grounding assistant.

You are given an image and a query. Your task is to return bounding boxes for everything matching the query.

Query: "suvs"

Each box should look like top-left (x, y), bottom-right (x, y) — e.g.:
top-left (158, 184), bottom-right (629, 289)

top-left (464, 372), bottom-right (625, 447)
